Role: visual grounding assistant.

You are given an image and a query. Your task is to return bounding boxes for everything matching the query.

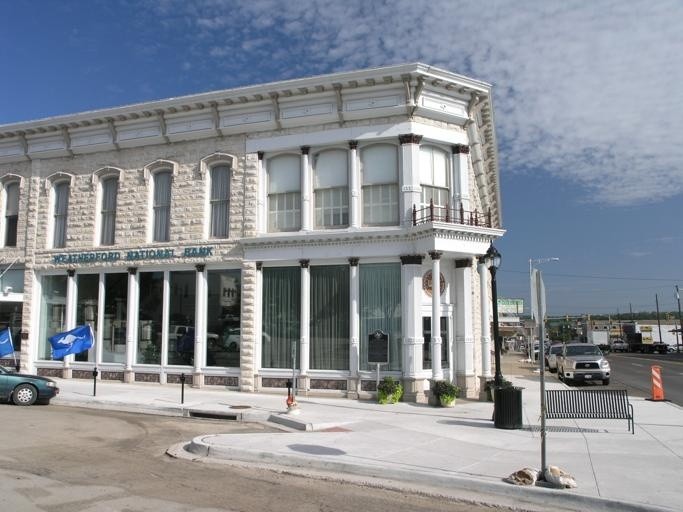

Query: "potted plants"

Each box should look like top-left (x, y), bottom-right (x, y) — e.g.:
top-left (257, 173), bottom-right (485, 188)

top-left (430, 380), bottom-right (461, 407)
top-left (377, 376), bottom-right (403, 404)
top-left (485, 379), bottom-right (512, 400)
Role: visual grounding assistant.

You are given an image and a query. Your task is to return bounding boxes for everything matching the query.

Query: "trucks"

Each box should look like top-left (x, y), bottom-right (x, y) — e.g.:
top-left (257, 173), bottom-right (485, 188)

top-left (625, 333), bottom-right (670, 355)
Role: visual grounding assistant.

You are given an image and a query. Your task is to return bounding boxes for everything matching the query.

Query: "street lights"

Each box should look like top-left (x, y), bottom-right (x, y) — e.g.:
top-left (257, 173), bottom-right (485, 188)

top-left (528, 257), bottom-right (567, 490)
top-left (481, 239), bottom-right (512, 420)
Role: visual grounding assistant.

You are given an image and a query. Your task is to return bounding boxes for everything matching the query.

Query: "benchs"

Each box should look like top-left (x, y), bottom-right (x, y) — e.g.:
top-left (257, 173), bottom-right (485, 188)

top-left (545, 389), bottom-right (634, 434)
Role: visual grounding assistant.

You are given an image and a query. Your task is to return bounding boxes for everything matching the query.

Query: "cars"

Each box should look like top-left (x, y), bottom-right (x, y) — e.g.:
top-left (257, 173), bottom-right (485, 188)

top-left (153, 324), bottom-right (271, 353)
top-left (521, 341), bottom-right (611, 386)
top-left (0, 364), bottom-right (59, 406)
top-left (667, 344), bottom-right (683, 352)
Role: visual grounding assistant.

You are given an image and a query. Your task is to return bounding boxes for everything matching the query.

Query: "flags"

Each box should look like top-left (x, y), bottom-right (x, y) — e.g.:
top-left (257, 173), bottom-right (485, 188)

top-left (48, 324), bottom-right (95, 360)
top-left (0, 330), bottom-right (14, 357)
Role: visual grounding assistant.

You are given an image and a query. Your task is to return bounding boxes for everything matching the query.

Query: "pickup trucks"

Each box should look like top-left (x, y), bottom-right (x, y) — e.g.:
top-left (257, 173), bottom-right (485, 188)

top-left (611, 339), bottom-right (628, 353)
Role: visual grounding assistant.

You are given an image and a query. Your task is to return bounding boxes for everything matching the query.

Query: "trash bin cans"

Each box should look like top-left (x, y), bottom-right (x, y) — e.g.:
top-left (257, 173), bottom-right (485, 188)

top-left (495, 390), bottom-right (522, 430)
